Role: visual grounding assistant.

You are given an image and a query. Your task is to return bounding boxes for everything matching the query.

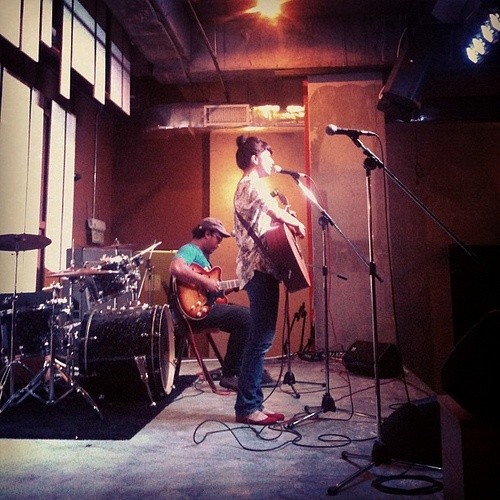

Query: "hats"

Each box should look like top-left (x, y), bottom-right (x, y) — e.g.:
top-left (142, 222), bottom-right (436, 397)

top-left (199, 217), bottom-right (231, 238)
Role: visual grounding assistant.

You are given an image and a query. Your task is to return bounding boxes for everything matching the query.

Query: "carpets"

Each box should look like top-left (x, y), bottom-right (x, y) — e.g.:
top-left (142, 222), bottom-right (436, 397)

top-left (0, 373), bottom-right (200, 441)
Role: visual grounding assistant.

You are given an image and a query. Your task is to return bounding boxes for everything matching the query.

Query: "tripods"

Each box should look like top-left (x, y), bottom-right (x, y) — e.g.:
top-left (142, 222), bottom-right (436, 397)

top-left (282, 180), bottom-right (378, 428)
top-left (0, 241), bottom-right (106, 419)
top-left (260, 287), bottom-right (326, 397)
top-left (330, 134), bottom-right (476, 496)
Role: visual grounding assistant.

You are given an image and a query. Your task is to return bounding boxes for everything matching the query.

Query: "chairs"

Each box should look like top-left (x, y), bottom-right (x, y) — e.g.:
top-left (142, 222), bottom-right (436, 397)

top-left (162, 277), bottom-right (226, 393)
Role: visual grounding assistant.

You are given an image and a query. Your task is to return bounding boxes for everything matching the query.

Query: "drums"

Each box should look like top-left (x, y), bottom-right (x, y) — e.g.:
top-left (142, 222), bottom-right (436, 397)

top-left (84, 254), bottom-right (141, 303)
top-left (79, 304), bottom-right (176, 398)
top-left (0, 298), bottom-right (74, 360)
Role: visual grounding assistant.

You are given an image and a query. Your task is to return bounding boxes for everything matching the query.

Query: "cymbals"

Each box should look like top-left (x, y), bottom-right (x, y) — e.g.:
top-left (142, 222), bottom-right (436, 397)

top-left (100, 243), bottom-right (137, 251)
top-left (47, 269), bottom-right (119, 277)
top-left (0, 234), bottom-right (51, 251)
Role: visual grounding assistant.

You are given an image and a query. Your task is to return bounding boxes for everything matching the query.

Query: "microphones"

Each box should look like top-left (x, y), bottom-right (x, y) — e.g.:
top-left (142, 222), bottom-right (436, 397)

top-left (325, 124), bottom-right (376, 137)
top-left (274, 165), bottom-right (306, 178)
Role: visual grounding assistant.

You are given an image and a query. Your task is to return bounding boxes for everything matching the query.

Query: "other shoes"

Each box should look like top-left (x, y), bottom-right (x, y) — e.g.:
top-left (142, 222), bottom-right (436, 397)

top-left (219, 377), bottom-right (239, 390)
top-left (236, 412), bottom-right (277, 424)
top-left (265, 412), bottom-right (285, 421)
top-left (261, 370), bottom-right (279, 387)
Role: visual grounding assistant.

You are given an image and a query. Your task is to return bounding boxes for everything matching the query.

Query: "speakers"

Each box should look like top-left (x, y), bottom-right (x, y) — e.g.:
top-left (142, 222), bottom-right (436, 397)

top-left (340, 339), bottom-right (401, 378)
top-left (377, 394), bottom-right (443, 460)
top-left (383, 31), bottom-right (440, 110)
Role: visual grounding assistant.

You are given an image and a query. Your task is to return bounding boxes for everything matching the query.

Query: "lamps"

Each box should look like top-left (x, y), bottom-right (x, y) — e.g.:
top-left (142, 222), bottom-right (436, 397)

top-left (465, 6), bottom-right (500, 63)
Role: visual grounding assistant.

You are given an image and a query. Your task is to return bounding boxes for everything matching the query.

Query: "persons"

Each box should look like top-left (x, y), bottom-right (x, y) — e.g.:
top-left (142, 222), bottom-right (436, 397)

top-left (169, 217), bottom-right (274, 389)
top-left (233, 134), bottom-right (306, 424)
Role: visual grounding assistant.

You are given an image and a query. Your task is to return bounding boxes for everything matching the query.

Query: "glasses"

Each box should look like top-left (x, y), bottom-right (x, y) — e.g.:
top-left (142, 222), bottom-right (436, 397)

top-left (211, 231), bottom-right (222, 243)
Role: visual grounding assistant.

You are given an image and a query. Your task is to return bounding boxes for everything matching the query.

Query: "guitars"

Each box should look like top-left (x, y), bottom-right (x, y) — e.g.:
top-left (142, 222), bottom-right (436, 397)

top-left (264, 188), bottom-right (311, 293)
top-left (172, 263), bottom-right (239, 320)
